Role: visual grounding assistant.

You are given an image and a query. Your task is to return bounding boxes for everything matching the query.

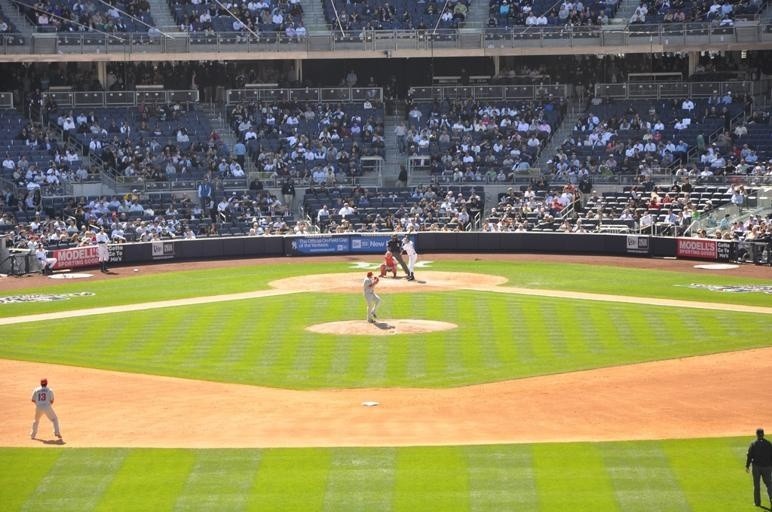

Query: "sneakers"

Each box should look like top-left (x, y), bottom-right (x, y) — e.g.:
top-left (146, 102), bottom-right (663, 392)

top-left (378, 271), bottom-right (415, 281)
top-left (369, 311), bottom-right (376, 323)
top-left (30, 435), bottom-right (35, 439)
top-left (55, 433), bottom-right (62, 439)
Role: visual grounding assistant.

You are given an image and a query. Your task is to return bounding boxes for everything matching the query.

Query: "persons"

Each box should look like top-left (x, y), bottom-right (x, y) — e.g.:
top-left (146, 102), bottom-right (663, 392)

top-left (362, 271), bottom-right (382, 324)
top-left (745, 428), bottom-right (771, 508)
top-left (1, 0), bottom-right (160, 47)
top-left (378, 250), bottom-right (399, 278)
top-left (2, 62), bottom-right (770, 262)
top-left (96, 226), bottom-right (110, 272)
top-left (386, 233), bottom-right (412, 279)
top-left (330, 0), bottom-right (473, 42)
top-left (628, 1), bottom-right (753, 37)
top-left (486, 0), bottom-right (608, 40)
top-left (166, 0), bottom-right (307, 42)
top-left (399, 236), bottom-right (419, 281)
top-left (36, 243), bottom-right (58, 275)
top-left (30, 378), bottom-right (62, 440)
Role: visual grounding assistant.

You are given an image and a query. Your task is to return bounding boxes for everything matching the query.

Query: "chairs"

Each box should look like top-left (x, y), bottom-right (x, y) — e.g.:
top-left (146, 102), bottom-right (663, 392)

top-left (0, 0), bottom-right (772, 244)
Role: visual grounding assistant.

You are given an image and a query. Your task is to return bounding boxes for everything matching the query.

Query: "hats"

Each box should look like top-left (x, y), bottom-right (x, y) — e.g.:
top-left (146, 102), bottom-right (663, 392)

top-left (367, 272), bottom-right (373, 277)
top-left (41, 378), bottom-right (48, 385)
top-left (755, 428), bottom-right (765, 438)
